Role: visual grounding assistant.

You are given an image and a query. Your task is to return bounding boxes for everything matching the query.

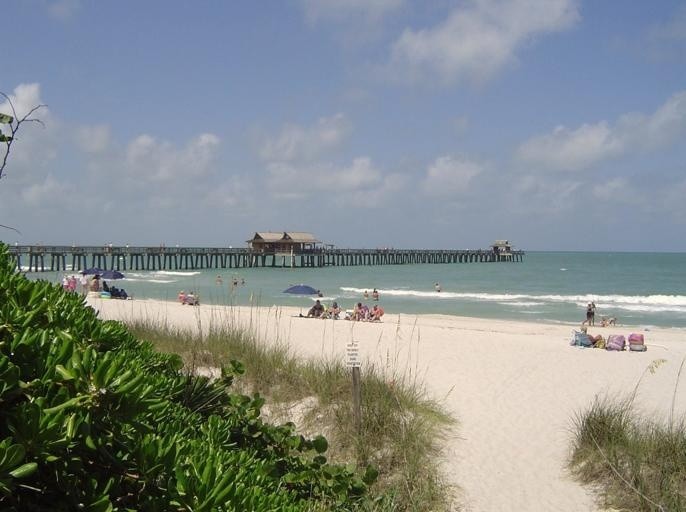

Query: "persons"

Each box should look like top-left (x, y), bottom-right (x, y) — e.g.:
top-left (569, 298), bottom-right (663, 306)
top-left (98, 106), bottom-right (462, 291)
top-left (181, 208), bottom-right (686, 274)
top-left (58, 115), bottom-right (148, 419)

top-left (62, 273), bottom-right (131, 301)
top-left (592, 303), bottom-right (598, 326)
top-left (232, 278), bottom-right (238, 286)
top-left (178, 291), bottom-right (188, 305)
top-left (373, 291), bottom-right (380, 301)
top-left (365, 304), bottom-right (380, 322)
top-left (240, 278), bottom-right (247, 286)
top-left (306, 300), bottom-right (324, 318)
top-left (363, 290), bottom-right (369, 299)
top-left (435, 283), bottom-right (441, 292)
top-left (320, 302), bottom-right (341, 319)
top-left (582, 302), bottom-right (592, 326)
top-left (186, 292), bottom-right (195, 306)
top-left (608, 317), bottom-right (617, 326)
top-left (193, 291), bottom-right (199, 306)
top-left (350, 302), bottom-right (364, 321)
top-left (372, 288), bottom-right (377, 297)
top-left (216, 275), bottom-right (223, 284)
top-left (580, 326), bottom-right (603, 344)
top-left (602, 319), bottom-right (607, 326)
top-left (318, 290), bottom-right (323, 297)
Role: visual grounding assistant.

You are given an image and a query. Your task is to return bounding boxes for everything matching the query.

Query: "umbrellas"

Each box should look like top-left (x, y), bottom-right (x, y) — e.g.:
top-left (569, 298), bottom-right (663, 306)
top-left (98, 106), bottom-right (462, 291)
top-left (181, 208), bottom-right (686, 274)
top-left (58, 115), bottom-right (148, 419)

top-left (282, 284), bottom-right (317, 314)
top-left (80, 267), bottom-right (104, 281)
top-left (101, 270), bottom-right (125, 287)
